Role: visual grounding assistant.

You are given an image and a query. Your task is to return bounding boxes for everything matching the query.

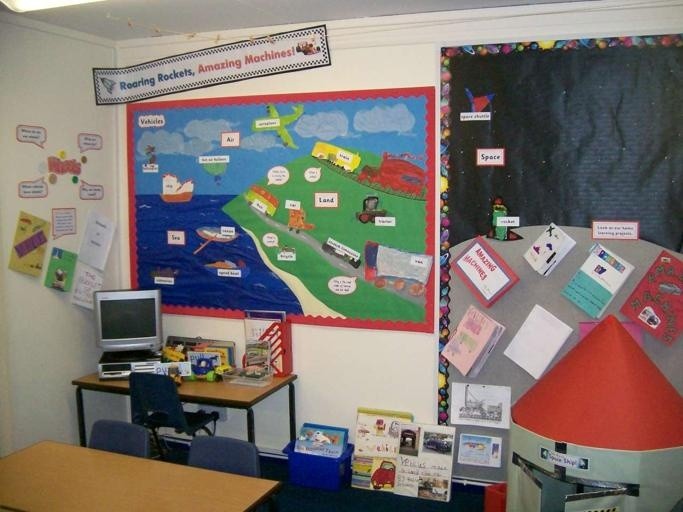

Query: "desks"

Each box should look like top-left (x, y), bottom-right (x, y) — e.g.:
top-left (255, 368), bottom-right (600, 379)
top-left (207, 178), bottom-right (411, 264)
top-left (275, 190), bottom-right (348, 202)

top-left (72, 367), bottom-right (298, 446)
top-left (0, 440), bottom-right (279, 511)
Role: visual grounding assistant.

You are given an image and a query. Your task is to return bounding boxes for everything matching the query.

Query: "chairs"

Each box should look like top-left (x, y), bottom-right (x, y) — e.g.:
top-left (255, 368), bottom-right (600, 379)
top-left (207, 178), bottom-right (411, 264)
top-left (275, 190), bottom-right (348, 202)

top-left (90, 420), bottom-right (263, 480)
top-left (128, 373), bottom-right (218, 458)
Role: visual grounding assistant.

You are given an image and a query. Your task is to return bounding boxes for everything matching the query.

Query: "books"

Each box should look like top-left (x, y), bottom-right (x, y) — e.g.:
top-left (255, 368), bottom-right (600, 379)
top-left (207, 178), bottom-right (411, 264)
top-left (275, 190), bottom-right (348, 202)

top-left (502, 305), bottom-right (573, 381)
top-left (449, 236), bottom-right (519, 307)
top-left (244, 308), bottom-right (286, 322)
top-left (243, 317), bottom-right (283, 375)
top-left (187, 338), bottom-right (236, 377)
top-left (440, 303), bottom-right (505, 380)
top-left (620, 248), bottom-right (683, 348)
top-left (559, 243), bottom-right (636, 319)
top-left (244, 338), bottom-right (271, 379)
top-left (522, 222), bottom-right (577, 276)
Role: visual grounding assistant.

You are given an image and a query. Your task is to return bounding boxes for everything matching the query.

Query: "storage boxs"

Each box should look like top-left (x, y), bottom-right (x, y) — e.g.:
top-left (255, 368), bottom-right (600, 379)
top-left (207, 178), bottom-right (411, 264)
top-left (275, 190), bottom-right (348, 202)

top-left (283, 439), bottom-right (353, 492)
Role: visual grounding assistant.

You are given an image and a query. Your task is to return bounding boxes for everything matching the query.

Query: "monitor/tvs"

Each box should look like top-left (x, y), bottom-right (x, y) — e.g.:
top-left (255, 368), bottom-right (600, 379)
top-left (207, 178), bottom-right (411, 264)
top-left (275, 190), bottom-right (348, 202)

top-left (93, 287), bottom-right (163, 353)
